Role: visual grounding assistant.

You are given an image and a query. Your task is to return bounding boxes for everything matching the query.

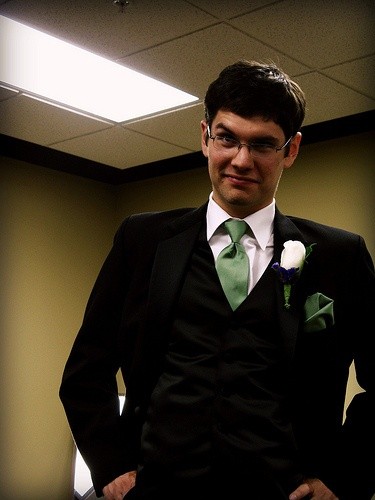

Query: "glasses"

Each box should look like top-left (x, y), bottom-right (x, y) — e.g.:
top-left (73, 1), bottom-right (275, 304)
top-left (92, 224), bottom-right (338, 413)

top-left (207, 125), bottom-right (292, 160)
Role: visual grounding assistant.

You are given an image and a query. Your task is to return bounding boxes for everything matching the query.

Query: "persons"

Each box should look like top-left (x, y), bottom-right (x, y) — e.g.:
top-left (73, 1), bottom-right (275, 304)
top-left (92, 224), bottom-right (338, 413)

top-left (58, 59), bottom-right (375, 500)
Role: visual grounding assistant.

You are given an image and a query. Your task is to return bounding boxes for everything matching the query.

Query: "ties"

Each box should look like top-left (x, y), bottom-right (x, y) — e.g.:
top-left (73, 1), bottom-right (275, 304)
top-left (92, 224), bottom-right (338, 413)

top-left (215, 219), bottom-right (249, 312)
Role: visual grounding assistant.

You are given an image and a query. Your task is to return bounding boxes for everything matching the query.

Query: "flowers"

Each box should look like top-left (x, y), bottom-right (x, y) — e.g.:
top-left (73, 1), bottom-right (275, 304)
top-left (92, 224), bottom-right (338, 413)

top-left (272, 240), bottom-right (317, 309)
